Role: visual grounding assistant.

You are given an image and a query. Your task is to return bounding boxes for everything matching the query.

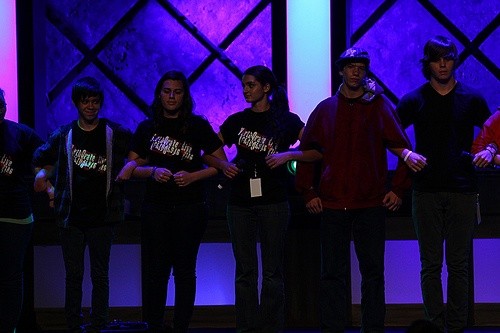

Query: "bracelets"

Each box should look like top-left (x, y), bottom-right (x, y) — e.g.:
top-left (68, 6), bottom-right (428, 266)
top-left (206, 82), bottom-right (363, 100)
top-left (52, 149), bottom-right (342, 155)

top-left (483, 144), bottom-right (497, 159)
top-left (49, 198), bottom-right (54, 203)
top-left (403, 151), bottom-right (412, 162)
top-left (151, 166), bottom-right (158, 180)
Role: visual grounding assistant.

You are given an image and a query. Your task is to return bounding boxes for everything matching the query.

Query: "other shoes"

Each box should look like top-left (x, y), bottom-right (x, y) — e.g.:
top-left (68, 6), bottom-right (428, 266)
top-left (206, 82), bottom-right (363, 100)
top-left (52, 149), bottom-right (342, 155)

top-left (17, 311), bottom-right (46, 333)
top-left (93, 320), bottom-right (120, 330)
top-left (65, 317), bottom-right (82, 333)
top-left (360, 328), bottom-right (383, 333)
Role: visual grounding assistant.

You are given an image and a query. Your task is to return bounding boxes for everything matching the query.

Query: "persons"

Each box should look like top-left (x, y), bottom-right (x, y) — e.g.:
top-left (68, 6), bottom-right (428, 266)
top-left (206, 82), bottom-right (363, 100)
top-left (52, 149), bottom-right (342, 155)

top-left (386, 36), bottom-right (500, 333)
top-left (201, 65), bottom-right (326, 332)
top-left (31, 77), bottom-right (151, 333)
top-left (295, 47), bottom-right (414, 333)
top-left (0, 89), bottom-right (60, 333)
top-left (127, 71), bottom-right (227, 333)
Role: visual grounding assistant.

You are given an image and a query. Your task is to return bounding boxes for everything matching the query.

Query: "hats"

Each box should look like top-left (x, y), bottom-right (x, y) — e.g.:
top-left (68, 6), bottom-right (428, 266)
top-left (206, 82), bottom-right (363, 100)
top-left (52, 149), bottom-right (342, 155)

top-left (336, 48), bottom-right (370, 64)
